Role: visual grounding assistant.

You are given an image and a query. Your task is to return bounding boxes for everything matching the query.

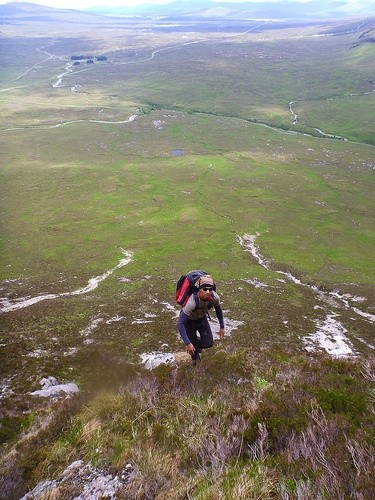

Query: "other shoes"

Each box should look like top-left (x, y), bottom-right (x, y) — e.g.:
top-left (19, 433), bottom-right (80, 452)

top-left (192, 339), bottom-right (202, 366)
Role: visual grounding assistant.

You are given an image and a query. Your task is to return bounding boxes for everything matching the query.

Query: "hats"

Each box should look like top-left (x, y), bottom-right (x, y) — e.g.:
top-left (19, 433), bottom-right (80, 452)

top-left (194, 275), bottom-right (214, 288)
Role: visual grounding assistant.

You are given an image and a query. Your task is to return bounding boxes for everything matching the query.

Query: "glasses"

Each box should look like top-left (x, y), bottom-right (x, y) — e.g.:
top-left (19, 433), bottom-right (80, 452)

top-left (202, 287), bottom-right (213, 292)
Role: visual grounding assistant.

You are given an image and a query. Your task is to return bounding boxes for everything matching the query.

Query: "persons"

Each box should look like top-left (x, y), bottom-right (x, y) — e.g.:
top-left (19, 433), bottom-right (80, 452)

top-left (176, 274), bottom-right (225, 367)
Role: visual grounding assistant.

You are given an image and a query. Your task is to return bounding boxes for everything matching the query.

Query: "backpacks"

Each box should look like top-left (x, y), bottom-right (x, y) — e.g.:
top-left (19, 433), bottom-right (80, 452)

top-left (176, 269), bottom-right (208, 307)
